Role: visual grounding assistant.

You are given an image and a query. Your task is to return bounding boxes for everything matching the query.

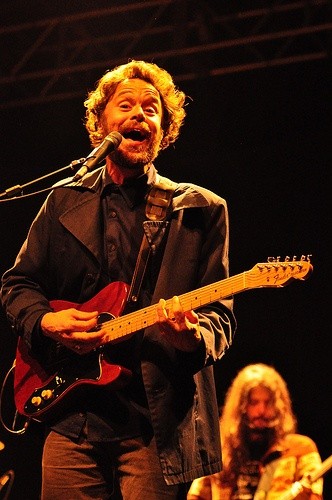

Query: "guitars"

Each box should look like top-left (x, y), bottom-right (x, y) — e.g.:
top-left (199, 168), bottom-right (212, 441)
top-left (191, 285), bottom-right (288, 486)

top-left (14, 253), bottom-right (314, 422)
top-left (266, 455), bottom-right (332, 500)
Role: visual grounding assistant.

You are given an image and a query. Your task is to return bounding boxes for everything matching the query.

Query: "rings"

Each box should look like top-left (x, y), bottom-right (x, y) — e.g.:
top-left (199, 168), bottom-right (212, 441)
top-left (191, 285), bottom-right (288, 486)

top-left (76, 344), bottom-right (82, 352)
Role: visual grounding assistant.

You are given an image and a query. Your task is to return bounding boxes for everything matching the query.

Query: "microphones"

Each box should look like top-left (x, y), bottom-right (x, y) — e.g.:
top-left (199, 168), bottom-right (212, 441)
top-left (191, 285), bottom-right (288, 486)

top-left (73, 130), bottom-right (122, 181)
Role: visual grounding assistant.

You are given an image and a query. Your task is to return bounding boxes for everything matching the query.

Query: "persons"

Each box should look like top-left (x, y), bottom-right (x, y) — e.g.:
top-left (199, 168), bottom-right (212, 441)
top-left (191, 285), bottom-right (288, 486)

top-left (0, 60), bottom-right (237, 500)
top-left (187, 365), bottom-right (326, 499)
